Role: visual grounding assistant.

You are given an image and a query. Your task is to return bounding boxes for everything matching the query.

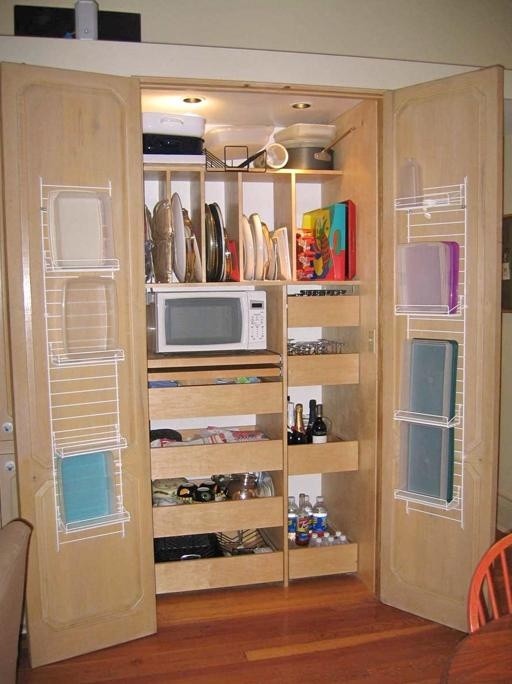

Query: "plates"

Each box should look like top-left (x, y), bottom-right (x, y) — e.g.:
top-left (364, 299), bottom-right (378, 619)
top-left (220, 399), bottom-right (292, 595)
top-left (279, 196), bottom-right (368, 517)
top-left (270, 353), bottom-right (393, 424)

top-left (147, 192), bottom-right (293, 282)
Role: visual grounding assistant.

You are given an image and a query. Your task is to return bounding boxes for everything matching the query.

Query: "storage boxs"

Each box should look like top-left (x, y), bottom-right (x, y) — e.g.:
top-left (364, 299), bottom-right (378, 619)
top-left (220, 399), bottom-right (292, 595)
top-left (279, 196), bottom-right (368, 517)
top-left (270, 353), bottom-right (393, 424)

top-left (402, 240), bottom-right (458, 503)
top-left (48, 189), bottom-right (116, 269)
top-left (62, 276), bottom-right (120, 361)
top-left (58, 450), bottom-right (116, 531)
top-left (142, 112), bottom-right (335, 164)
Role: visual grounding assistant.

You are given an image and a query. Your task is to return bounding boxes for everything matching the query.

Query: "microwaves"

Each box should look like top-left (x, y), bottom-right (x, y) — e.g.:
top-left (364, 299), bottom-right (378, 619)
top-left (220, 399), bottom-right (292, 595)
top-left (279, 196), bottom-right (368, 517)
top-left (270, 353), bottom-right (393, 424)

top-left (147, 287), bottom-right (269, 354)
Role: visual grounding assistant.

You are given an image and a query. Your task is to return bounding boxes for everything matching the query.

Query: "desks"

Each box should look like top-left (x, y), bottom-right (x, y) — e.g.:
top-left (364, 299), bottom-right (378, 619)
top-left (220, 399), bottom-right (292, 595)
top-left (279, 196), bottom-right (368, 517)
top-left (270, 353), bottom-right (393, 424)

top-left (435, 608), bottom-right (512, 682)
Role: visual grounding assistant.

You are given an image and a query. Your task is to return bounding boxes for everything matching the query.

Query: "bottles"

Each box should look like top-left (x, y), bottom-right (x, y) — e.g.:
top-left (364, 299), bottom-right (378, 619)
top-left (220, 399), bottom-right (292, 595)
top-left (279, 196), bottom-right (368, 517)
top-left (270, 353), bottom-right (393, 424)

top-left (289, 493), bottom-right (351, 548)
top-left (288, 395), bottom-right (331, 444)
top-left (401, 157), bottom-right (423, 206)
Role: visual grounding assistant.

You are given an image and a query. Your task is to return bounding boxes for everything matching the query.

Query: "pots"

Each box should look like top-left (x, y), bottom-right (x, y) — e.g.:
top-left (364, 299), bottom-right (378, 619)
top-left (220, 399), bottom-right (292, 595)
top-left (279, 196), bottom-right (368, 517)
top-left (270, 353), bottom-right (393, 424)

top-left (284, 125), bottom-right (358, 168)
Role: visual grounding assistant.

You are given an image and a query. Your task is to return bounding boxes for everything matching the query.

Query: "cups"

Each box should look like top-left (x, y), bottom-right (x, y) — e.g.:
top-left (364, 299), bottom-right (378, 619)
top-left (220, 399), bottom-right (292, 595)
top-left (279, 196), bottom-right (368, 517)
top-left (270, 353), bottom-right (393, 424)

top-left (253, 144), bottom-right (288, 170)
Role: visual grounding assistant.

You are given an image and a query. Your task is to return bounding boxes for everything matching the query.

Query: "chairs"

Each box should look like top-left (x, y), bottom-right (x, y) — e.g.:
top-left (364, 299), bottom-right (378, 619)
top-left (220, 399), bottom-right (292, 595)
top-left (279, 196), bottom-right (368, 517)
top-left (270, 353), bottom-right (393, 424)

top-left (1, 519), bottom-right (33, 683)
top-left (464, 525), bottom-right (512, 630)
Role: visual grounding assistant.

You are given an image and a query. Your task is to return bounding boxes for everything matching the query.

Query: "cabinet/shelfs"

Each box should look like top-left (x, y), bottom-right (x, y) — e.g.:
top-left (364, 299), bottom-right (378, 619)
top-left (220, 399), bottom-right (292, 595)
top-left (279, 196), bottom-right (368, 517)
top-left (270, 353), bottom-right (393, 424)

top-left (396, 155), bottom-right (466, 517)
top-left (146, 284), bottom-right (283, 595)
top-left (144, 165), bottom-right (361, 288)
top-left (43, 177), bottom-right (131, 552)
top-left (287, 280), bottom-right (361, 580)
